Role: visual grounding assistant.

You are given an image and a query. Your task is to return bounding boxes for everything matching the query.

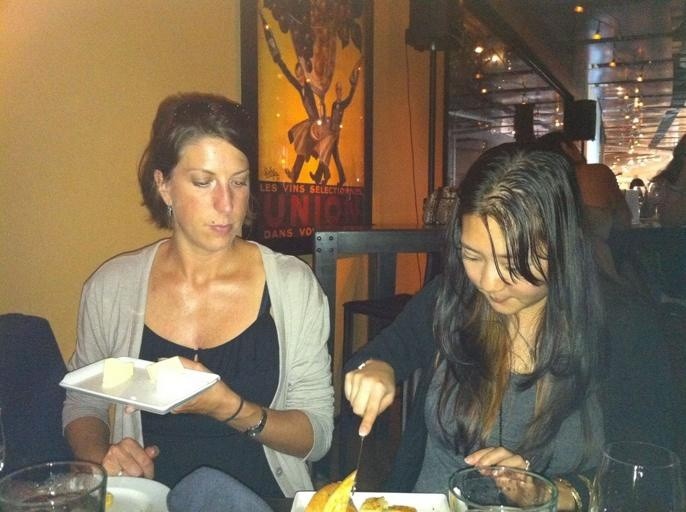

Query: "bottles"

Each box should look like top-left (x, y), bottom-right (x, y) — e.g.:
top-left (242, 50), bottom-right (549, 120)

top-left (259, 12), bottom-right (282, 63)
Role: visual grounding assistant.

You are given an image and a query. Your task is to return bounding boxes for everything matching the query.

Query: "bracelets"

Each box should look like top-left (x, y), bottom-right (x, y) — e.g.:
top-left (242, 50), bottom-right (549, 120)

top-left (217, 391), bottom-right (243, 424)
top-left (553, 473), bottom-right (599, 512)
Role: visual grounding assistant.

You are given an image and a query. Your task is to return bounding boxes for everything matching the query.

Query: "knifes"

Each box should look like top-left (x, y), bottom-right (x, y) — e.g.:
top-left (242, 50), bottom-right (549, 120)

top-left (351, 412), bottom-right (366, 496)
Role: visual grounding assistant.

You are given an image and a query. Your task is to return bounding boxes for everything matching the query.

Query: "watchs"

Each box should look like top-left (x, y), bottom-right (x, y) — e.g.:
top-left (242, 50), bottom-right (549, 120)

top-left (241, 405), bottom-right (267, 440)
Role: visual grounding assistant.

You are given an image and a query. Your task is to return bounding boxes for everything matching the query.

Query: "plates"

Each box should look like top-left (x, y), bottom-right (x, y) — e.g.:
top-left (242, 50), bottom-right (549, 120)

top-left (56, 355), bottom-right (221, 414)
top-left (290, 492), bottom-right (449, 511)
top-left (55, 473), bottom-right (170, 512)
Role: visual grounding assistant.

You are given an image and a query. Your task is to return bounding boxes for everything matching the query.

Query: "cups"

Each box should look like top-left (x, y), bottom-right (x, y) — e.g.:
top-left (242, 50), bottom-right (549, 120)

top-left (449, 439), bottom-right (684, 511)
top-left (0, 461), bottom-right (106, 511)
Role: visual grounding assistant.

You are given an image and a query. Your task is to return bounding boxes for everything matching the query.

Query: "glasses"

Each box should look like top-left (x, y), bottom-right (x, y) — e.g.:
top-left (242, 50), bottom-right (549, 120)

top-left (164, 99), bottom-right (252, 133)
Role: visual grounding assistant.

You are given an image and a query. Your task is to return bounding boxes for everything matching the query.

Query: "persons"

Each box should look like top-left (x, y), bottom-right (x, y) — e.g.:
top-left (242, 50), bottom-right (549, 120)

top-left (344, 143), bottom-right (676, 511)
top-left (534, 132), bottom-right (686, 291)
top-left (276, 57), bottom-right (357, 188)
top-left (60, 93), bottom-right (335, 498)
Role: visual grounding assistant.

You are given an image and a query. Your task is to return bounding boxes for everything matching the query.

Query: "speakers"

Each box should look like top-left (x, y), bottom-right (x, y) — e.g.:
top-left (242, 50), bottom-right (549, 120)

top-left (405, 0), bottom-right (464, 51)
top-left (514, 104), bottom-right (534, 144)
top-left (564, 100), bottom-right (596, 141)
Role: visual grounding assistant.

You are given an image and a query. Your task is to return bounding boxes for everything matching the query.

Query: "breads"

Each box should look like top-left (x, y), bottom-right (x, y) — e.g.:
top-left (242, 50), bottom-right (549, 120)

top-left (306, 470), bottom-right (415, 512)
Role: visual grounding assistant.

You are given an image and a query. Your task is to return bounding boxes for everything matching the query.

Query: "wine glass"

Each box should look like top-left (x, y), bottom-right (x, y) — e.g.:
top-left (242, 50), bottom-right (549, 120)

top-left (288, 11), bottom-right (339, 142)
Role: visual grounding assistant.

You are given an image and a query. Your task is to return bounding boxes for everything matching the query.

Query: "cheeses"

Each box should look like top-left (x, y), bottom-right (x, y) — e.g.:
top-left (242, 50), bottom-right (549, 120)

top-left (102, 358), bottom-right (133, 389)
top-left (147, 356), bottom-right (183, 381)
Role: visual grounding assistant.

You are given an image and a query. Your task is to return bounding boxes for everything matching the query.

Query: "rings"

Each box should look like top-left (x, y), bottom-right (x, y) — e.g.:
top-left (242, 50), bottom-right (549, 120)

top-left (117, 468), bottom-right (125, 476)
top-left (525, 458), bottom-right (531, 471)
